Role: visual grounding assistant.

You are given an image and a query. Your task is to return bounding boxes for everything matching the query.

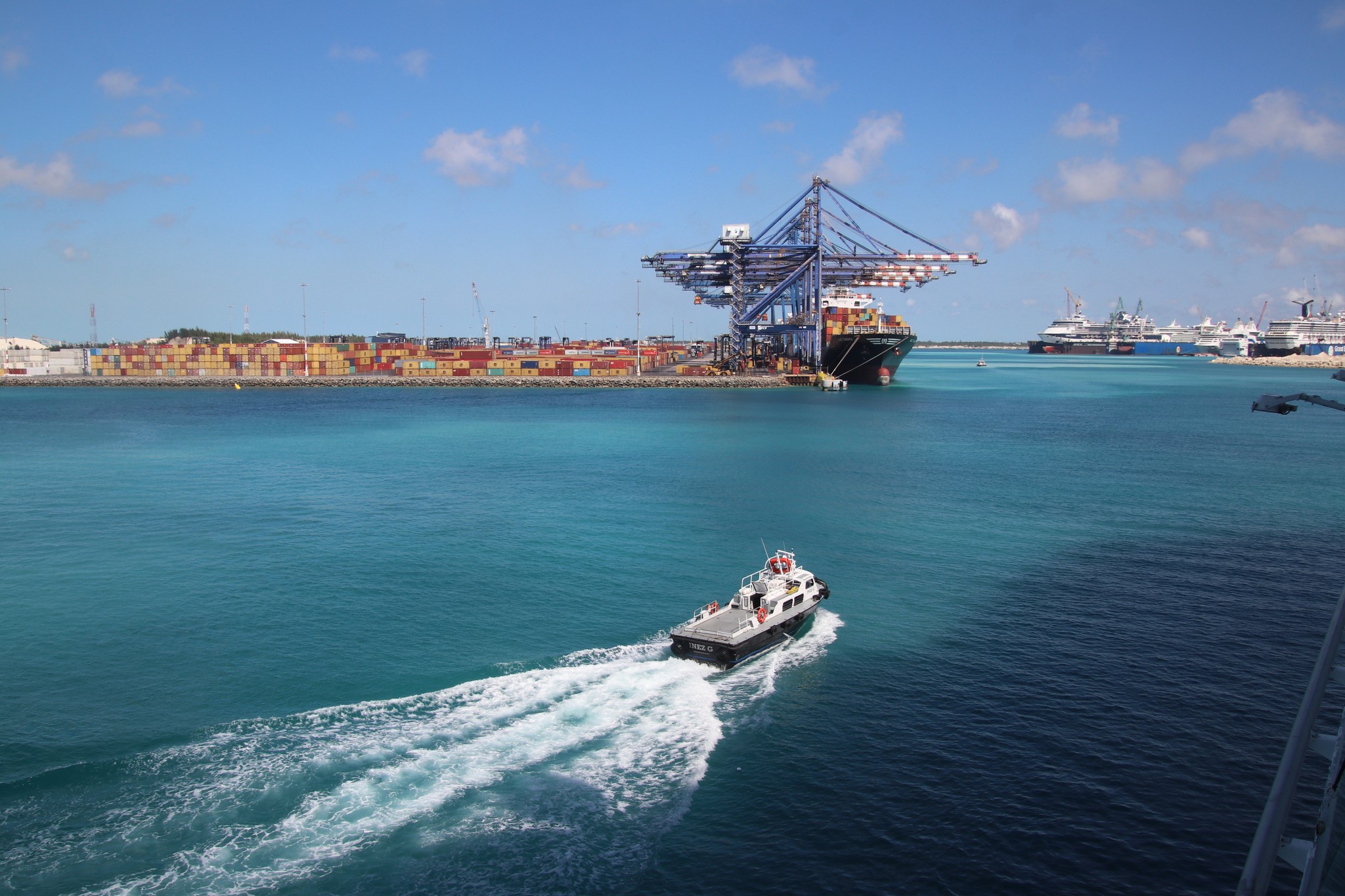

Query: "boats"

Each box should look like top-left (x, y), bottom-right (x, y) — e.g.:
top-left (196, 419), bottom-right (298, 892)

top-left (668, 536), bottom-right (831, 670)
top-left (821, 296), bottom-right (918, 386)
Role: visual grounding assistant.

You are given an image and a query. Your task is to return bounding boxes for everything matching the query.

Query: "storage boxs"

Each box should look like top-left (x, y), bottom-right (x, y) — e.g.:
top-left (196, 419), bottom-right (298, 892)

top-left (0, 304), bottom-right (908, 377)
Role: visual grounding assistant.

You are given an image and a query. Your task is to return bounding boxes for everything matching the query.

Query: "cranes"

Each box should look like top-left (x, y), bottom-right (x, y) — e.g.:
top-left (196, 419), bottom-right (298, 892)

top-left (472, 281), bottom-right (485, 336)
top-left (640, 175), bottom-right (987, 368)
top-left (1108, 296), bottom-right (1144, 330)
top-left (1064, 286), bottom-right (1082, 318)
top-left (1303, 274), bottom-right (1326, 317)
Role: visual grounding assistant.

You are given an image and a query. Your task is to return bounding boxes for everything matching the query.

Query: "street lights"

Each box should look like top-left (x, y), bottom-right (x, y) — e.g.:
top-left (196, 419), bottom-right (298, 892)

top-left (396, 323), bottom-right (399, 338)
top-left (615, 326), bottom-right (618, 345)
top-left (300, 283), bottom-right (309, 376)
top-left (421, 297), bottom-right (427, 345)
top-left (227, 305), bottom-right (234, 344)
top-left (322, 310), bottom-right (326, 343)
top-left (689, 321), bottom-right (693, 344)
top-left (533, 315), bottom-right (537, 349)
top-left (634, 279), bottom-right (642, 377)
top-left (489, 310), bottom-right (496, 349)
top-left (440, 325), bottom-right (442, 343)
top-left (535, 317), bottom-right (539, 347)
top-left (514, 325), bottom-right (516, 347)
top-left (562, 320), bottom-right (566, 337)
top-left (469, 326), bottom-right (471, 339)
top-left (584, 322), bottom-right (588, 345)
top-left (0, 287), bottom-right (12, 378)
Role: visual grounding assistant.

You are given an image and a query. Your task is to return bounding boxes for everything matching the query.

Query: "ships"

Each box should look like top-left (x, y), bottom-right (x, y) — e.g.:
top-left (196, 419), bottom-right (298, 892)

top-left (1030, 299), bottom-right (1345, 356)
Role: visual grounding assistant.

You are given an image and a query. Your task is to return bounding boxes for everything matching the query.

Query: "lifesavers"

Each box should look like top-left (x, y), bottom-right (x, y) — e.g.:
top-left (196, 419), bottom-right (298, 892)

top-left (770, 625), bottom-right (778, 636)
top-left (758, 608), bottom-right (766, 623)
top-left (824, 590), bottom-right (830, 599)
top-left (784, 620), bottom-right (792, 630)
top-left (796, 613), bottom-right (805, 622)
top-left (710, 602), bottom-right (719, 613)
top-left (819, 588), bottom-right (828, 595)
top-left (812, 595), bottom-right (822, 598)
top-left (713, 650), bottom-right (730, 665)
top-left (725, 602), bottom-right (730, 606)
top-left (696, 617), bottom-right (700, 620)
top-left (670, 643), bottom-right (684, 657)
